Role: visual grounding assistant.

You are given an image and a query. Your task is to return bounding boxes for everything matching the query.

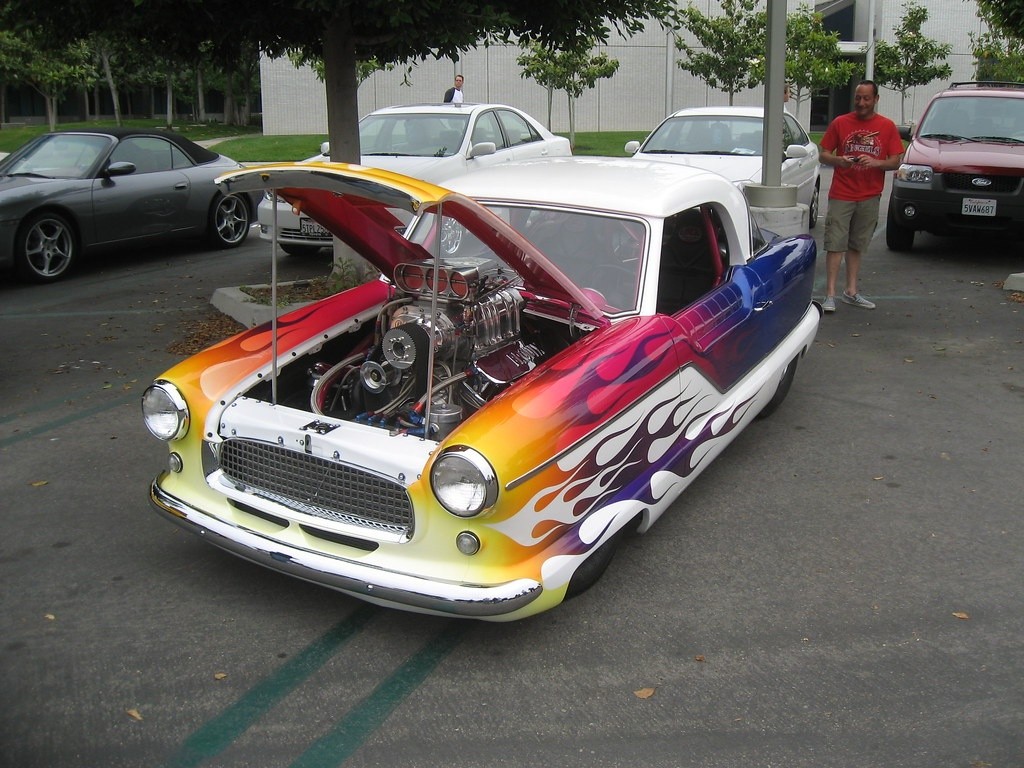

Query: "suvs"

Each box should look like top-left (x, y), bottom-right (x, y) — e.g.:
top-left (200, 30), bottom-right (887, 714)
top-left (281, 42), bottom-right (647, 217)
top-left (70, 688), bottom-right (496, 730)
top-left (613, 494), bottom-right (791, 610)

top-left (884, 79), bottom-right (1024, 253)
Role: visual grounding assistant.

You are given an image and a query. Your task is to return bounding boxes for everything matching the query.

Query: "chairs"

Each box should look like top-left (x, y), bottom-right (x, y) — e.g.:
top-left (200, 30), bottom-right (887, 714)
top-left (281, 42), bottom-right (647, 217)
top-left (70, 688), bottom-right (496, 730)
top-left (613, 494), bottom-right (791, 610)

top-left (472, 128), bottom-right (489, 144)
top-left (659, 207), bottom-right (717, 314)
top-left (440, 128), bottom-right (462, 150)
top-left (706, 123), bottom-right (732, 146)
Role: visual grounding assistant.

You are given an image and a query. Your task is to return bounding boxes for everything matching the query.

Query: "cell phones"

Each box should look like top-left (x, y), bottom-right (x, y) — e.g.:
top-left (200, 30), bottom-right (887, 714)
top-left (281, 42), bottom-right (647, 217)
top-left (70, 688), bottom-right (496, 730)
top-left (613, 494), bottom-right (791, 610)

top-left (848, 157), bottom-right (860, 162)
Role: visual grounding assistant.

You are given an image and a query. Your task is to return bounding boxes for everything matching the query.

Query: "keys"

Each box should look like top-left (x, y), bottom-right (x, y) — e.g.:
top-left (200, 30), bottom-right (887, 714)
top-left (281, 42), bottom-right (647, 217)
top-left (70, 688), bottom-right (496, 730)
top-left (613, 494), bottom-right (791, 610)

top-left (849, 157), bottom-right (860, 162)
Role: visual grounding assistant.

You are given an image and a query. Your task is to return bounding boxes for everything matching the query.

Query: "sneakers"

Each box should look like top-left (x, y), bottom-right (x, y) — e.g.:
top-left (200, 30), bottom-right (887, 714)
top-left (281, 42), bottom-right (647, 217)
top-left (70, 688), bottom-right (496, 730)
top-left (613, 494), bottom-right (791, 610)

top-left (822, 295), bottom-right (836, 311)
top-left (842, 289), bottom-right (875, 309)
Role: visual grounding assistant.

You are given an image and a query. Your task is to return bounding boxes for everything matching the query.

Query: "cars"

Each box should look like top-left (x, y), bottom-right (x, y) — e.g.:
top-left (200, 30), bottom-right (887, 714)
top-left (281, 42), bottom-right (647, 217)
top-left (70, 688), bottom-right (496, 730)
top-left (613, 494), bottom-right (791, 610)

top-left (138, 154), bottom-right (826, 626)
top-left (0, 126), bottom-right (263, 286)
top-left (623, 104), bottom-right (822, 244)
top-left (254, 103), bottom-right (573, 257)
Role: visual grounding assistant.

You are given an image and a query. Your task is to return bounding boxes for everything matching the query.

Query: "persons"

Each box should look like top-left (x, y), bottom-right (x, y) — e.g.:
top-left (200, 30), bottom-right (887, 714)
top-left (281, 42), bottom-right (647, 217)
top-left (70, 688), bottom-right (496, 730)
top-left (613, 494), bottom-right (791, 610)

top-left (818, 80), bottom-right (905, 312)
top-left (784, 83), bottom-right (791, 113)
top-left (444, 75), bottom-right (465, 129)
